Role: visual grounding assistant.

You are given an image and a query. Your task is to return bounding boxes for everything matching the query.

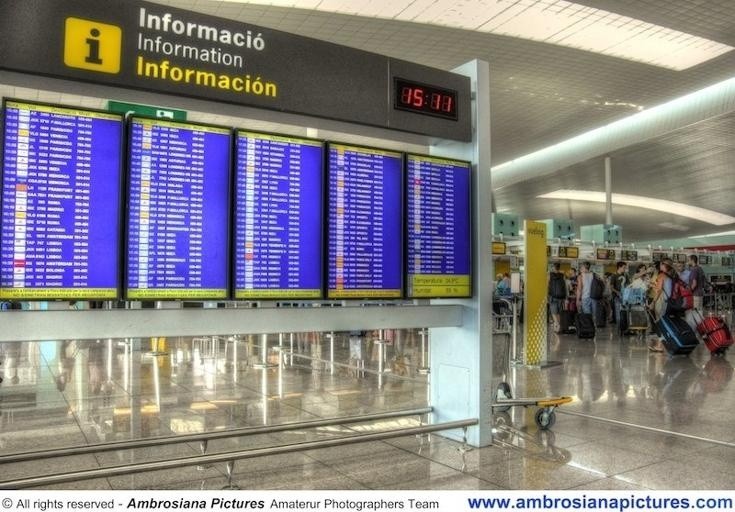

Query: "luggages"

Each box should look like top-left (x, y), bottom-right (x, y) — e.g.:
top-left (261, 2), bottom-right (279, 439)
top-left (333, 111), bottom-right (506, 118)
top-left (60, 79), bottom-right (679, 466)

top-left (595, 305), bottom-right (606, 328)
top-left (648, 308), bottom-right (734, 357)
top-left (619, 303), bottom-right (651, 337)
top-left (560, 303), bottom-right (596, 339)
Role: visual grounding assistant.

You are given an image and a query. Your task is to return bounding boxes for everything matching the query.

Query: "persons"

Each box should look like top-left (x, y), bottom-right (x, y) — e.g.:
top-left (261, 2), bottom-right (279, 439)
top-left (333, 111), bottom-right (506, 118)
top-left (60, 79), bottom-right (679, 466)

top-left (495, 255), bottom-right (705, 352)
top-left (5, 329), bottom-right (427, 393)
top-left (705, 278), bottom-right (735, 310)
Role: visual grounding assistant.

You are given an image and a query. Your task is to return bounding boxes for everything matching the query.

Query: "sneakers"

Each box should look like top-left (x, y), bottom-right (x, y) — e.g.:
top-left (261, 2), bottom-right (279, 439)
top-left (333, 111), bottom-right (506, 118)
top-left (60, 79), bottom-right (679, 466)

top-left (553, 321), bottom-right (562, 334)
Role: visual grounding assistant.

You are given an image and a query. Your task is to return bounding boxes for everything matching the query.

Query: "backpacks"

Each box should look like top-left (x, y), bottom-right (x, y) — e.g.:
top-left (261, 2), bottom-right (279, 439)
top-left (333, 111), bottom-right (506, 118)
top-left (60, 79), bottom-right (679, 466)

top-left (552, 272), bottom-right (567, 299)
top-left (589, 273), bottom-right (605, 299)
top-left (658, 270), bottom-right (694, 311)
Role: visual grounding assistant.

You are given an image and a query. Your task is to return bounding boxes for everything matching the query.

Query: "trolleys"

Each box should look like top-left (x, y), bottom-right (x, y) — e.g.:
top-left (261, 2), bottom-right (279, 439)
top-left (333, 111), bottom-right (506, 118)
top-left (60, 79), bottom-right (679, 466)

top-left (492, 296), bottom-right (572, 430)
